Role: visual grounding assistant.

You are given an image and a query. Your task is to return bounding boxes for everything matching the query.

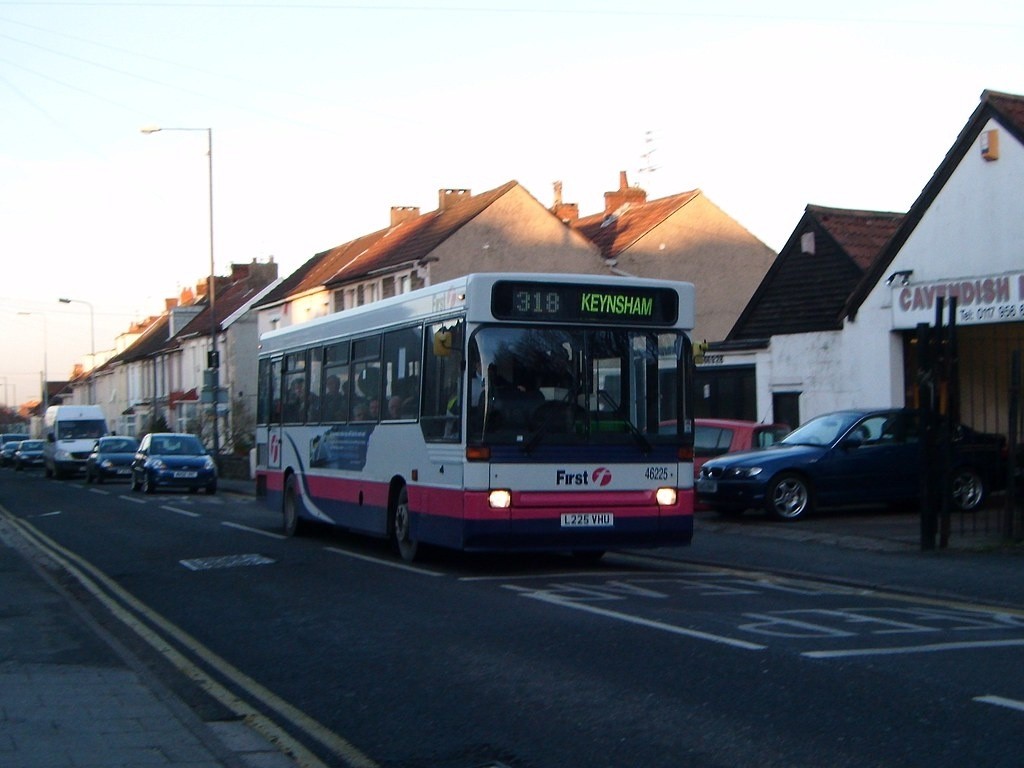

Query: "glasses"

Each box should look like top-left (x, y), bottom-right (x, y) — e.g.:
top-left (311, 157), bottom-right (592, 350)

top-left (353, 413), bottom-right (369, 418)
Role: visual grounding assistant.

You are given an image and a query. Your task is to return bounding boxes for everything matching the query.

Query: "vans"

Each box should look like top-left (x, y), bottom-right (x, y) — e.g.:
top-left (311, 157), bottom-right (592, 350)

top-left (43, 403), bottom-right (116, 479)
top-left (641, 418), bottom-right (792, 508)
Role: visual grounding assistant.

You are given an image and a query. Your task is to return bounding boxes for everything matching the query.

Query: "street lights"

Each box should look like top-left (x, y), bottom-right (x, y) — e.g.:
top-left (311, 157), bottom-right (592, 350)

top-left (58, 298), bottom-right (96, 405)
top-left (19, 311), bottom-right (48, 413)
top-left (142, 128), bottom-right (219, 465)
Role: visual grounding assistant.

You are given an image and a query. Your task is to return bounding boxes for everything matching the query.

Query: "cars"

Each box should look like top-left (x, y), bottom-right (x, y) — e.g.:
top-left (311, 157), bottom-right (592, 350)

top-left (131, 433), bottom-right (218, 495)
top-left (0, 442), bottom-right (20, 464)
top-left (88, 437), bottom-right (140, 483)
top-left (14, 439), bottom-right (45, 471)
top-left (698, 407), bottom-right (1008, 521)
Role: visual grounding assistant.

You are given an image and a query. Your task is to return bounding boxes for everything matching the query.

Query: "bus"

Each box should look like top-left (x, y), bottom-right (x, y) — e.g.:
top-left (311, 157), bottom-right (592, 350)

top-left (251, 273), bottom-right (709, 563)
top-left (0, 433), bottom-right (30, 446)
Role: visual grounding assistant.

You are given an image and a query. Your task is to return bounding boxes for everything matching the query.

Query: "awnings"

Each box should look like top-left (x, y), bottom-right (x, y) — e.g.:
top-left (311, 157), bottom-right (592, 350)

top-left (173, 387), bottom-right (199, 402)
top-left (122, 407), bottom-right (135, 416)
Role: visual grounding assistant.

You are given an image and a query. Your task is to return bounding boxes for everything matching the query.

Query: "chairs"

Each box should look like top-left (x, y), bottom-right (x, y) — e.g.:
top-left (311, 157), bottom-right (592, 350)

top-left (353, 398), bottom-right (372, 420)
top-left (857, 425), bottom-right (871, 447)
top-left (400, 396), bottom-right (416, 419)
top-left (879, 418), bottom-right (901, 443)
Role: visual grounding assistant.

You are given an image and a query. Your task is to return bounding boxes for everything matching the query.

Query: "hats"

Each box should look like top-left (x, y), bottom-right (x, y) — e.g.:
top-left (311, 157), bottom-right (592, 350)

top-left (328, 376), bottom-right (340, 385)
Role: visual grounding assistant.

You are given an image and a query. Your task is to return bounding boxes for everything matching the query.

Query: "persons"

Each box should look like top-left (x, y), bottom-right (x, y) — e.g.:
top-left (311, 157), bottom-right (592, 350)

top-left (285, 358), bottom-right (546, 439)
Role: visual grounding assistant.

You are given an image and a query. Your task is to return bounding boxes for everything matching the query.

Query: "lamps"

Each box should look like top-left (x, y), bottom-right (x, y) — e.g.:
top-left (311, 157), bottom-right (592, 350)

top-left (886, 270), bottom-right (913, 287)
